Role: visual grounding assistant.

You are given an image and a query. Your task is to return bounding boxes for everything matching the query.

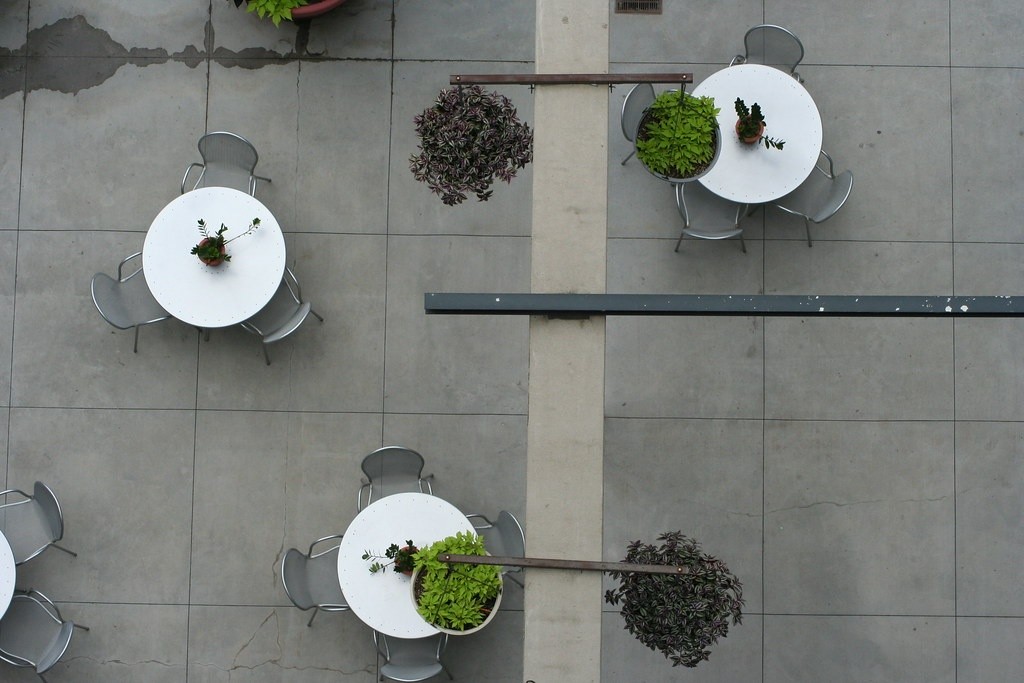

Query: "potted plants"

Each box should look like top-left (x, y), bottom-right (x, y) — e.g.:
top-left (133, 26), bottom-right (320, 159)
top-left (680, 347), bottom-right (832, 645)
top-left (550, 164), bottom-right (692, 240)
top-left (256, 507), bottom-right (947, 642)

top-left (191, 217), bottom-right (262, 267)
top-left (409, 530), bottom-right (503, 636)
top-left (228, 0), bottom-right (345, 30)
top-left (361, 539), bottom-right (419, 576)
top-left (735, 97), bottom-right (786, 150)
top-left (632, 88), bottom-right (722, 183)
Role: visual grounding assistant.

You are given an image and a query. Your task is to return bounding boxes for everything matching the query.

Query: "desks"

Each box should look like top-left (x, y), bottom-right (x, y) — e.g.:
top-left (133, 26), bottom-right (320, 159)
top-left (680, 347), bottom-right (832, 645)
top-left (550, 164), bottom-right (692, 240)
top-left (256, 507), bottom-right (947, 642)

top-left (336, 491), bottom-right (479, 639)
top-left (142, 185), bottom-right (287, 342)
top-left (0, 531), bottom-right (16, 619)
top-left (691, 64), bottom-right (823, 203)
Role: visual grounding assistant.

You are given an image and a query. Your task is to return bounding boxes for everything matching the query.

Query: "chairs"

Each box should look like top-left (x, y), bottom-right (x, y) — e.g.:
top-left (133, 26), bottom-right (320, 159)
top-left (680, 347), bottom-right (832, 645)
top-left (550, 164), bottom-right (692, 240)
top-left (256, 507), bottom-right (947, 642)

top-left (466, 510), bottom-right (525, 588)
top-left (373, 630), bottom-right (454, 683)
top-left (180, 130), bottom-right (272, 197)
top-left (237, 266), bottom-right (324, 366)
top-left (0, 588), bottom-right (89, 683)
top-left (670, 183), bottom-right (747, 254)
top-left (89, 251), bottom-right (203, 353)
top-left (356, 445), bottom-right (434, 513)
top-left (728, 22), bottom-right (804, 83)
top-left (0, 480), bottom-right (78, 566)
top-left (747, 149), bottom-right (853, 247)
top-left (281, 534), bottom-right (350, 627)
top-left (621, 83), bottom-right (690, 166)
